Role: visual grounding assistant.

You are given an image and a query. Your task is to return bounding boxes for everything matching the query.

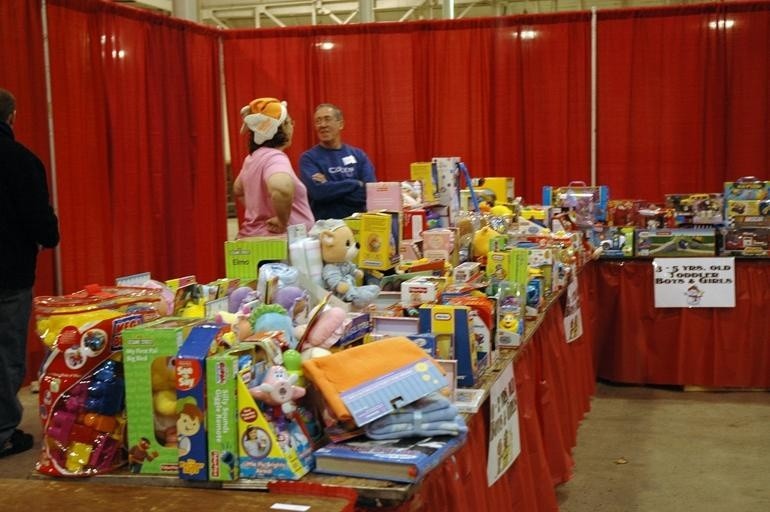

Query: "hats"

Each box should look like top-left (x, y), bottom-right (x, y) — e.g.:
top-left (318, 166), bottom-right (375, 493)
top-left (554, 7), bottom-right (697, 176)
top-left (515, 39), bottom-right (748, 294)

top-left (239, 97), bottom-right (288, 145)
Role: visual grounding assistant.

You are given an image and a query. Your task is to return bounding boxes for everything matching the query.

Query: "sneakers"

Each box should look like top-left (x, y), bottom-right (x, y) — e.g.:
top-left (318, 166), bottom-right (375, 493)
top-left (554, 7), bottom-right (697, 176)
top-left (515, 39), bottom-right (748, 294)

top-left (0, 428), bottom-right (34, 456)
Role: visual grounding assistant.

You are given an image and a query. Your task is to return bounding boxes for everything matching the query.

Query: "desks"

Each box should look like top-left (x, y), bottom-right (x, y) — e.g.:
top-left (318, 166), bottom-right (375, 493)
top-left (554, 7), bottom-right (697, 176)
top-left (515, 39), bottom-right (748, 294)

top-left (0, 251), bottom-right (770, 512)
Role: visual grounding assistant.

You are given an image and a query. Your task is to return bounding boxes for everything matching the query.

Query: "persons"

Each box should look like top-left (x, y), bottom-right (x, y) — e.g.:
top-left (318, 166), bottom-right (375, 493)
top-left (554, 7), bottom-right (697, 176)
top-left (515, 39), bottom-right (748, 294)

top-left (231, 95), bottom-right (316, 241)
top-left (298, 101), bottom-right (378, 221)
top-left (0, 86), bottom-right (61, 459)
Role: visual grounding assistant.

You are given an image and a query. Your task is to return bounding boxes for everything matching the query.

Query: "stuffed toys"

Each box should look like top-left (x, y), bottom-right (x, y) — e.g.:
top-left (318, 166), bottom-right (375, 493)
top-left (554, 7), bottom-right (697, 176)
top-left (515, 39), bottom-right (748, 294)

top-left (228, 286), bottom-right (310, 338)
top-left (320, 222), bottom-right (382, 307)
